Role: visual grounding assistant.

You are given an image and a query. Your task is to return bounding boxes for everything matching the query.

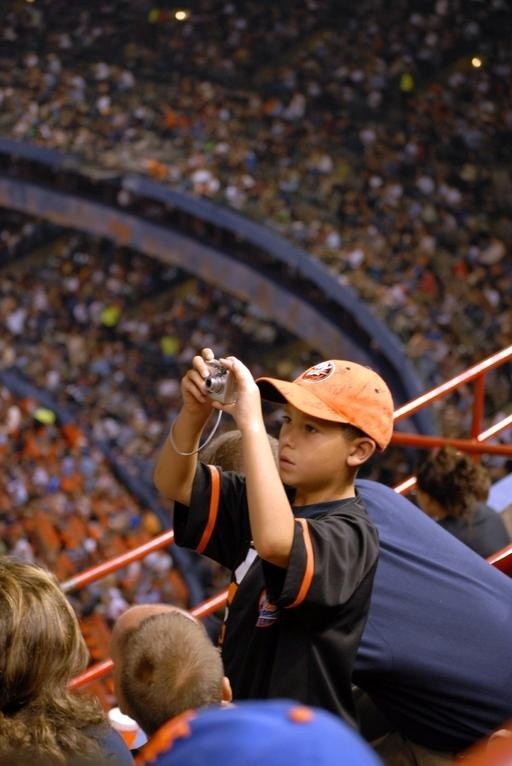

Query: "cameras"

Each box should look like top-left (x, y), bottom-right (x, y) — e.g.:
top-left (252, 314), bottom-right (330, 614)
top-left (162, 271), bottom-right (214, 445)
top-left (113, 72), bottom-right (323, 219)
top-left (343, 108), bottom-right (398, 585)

top-left (200, 359), bottom-right (234, 404)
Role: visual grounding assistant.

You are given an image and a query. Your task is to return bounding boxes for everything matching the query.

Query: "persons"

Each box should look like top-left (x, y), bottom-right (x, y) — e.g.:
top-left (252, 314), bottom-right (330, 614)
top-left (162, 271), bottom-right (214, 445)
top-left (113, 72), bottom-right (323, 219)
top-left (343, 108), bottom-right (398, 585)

top-left (154, 349), bottom-right (393, 720)
top-left (118, 610), bottom-right (382, 765)
top-left (204, 429), bottom-right (512, 764)
top-left (0, 1), bottom-right (510, 433)
top-left (1, 557), bottom-right (135, 766)
top-left (0, 431), bottom-right (191, 713)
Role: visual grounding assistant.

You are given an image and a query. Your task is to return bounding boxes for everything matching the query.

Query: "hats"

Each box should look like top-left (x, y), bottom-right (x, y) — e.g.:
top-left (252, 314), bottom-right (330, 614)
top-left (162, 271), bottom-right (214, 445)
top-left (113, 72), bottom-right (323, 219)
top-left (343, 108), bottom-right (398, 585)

top-left (253, 353), bottom-right (396, 455)
